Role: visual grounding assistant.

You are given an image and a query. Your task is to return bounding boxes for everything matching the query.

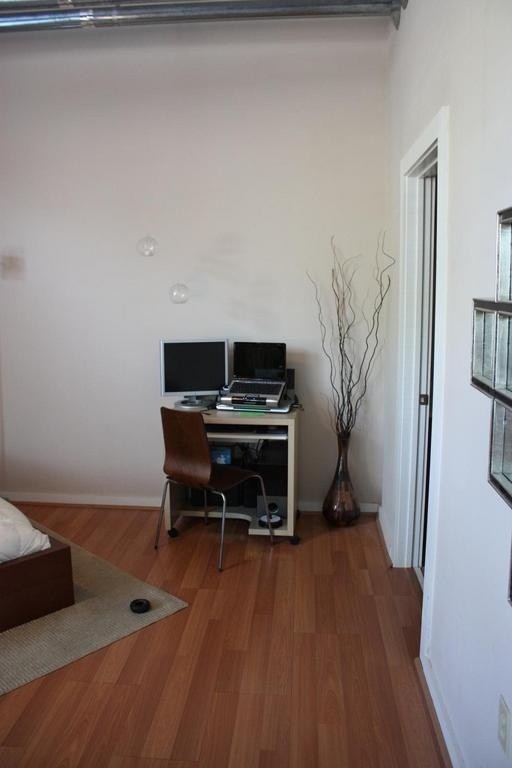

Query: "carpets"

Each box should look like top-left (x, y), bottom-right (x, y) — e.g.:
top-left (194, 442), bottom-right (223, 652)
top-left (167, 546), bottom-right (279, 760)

top-left (0, 518), bottom-right (190, 696)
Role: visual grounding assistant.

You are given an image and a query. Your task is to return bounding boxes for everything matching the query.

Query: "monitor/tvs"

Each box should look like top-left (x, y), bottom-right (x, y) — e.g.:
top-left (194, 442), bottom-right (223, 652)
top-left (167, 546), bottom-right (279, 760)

top-left (160, 337), bottom-right (228, 411)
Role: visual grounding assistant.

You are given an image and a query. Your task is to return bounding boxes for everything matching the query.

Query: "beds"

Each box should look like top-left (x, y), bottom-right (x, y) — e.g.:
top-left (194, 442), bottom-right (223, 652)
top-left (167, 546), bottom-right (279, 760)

top-left (0, 498), bottom-right (75, 635)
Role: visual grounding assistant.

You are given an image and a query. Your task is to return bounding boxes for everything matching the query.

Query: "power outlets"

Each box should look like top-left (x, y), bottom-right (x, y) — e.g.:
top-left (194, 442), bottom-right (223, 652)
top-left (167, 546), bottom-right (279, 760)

top-left (497, 694), bottom-right (510, 753)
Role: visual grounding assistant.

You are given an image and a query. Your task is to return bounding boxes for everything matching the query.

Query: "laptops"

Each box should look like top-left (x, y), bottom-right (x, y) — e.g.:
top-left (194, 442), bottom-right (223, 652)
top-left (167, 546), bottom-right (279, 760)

top-left (219, 341), bottom-right (287, 399)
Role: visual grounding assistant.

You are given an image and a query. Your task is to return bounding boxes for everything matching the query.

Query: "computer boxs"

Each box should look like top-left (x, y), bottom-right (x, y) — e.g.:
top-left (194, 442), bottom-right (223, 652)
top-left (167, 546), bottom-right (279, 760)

top-left (255, 441), bottom-right (287, 517)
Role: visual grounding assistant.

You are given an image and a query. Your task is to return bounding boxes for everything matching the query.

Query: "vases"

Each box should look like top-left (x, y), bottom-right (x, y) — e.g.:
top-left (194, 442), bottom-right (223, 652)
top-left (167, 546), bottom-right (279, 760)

top-left (320, 430), bottom-right (359, 528)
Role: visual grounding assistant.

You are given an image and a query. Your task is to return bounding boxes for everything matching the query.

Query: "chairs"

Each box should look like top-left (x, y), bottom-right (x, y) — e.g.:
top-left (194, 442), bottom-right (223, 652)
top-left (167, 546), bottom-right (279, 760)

top-left (153, 406), bottom-right (275, 572)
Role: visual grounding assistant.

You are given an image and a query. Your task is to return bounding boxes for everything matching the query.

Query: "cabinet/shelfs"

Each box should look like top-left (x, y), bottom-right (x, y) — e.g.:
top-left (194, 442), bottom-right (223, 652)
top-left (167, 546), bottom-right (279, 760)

top-left (469, 205), bottom-right (512, 510)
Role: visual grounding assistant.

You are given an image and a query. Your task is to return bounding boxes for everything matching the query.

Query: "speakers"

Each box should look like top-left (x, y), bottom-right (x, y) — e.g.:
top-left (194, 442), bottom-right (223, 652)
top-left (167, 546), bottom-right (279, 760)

top-left (286, 367), bottom-right (295, 390)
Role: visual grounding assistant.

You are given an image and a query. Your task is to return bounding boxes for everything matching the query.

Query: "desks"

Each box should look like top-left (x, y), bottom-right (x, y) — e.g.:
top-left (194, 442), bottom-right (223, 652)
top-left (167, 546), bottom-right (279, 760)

top-left (162, 404), bottom-right (301, 546)
